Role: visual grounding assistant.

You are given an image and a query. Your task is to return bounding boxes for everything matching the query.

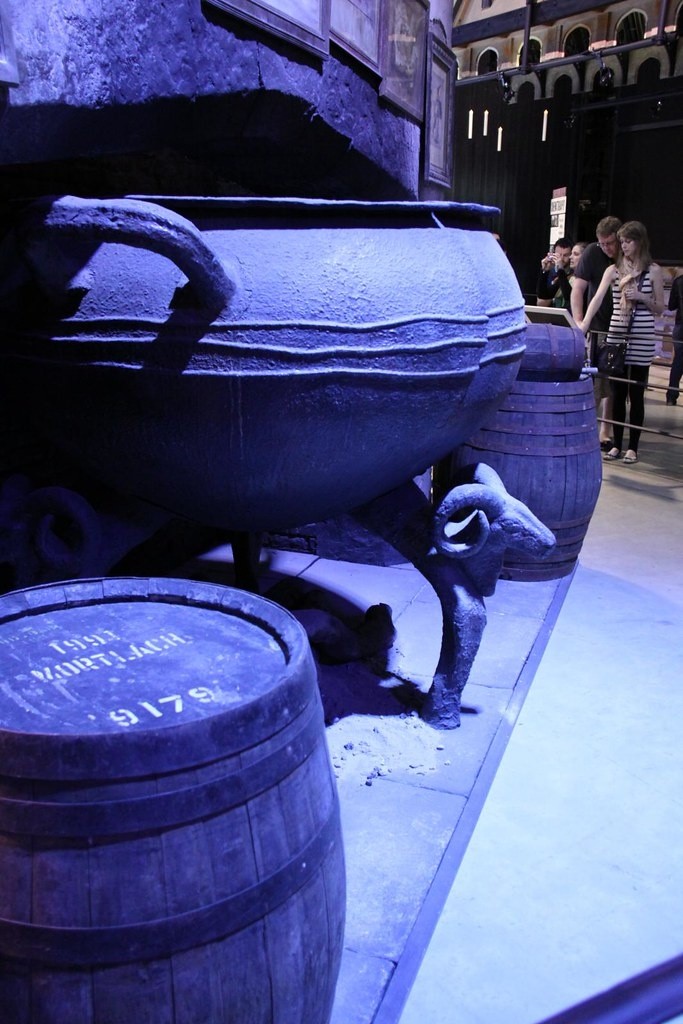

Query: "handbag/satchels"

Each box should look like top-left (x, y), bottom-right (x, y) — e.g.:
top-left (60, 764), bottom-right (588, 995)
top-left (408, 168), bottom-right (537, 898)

top-left (599, 342), bottom-right (626, 377)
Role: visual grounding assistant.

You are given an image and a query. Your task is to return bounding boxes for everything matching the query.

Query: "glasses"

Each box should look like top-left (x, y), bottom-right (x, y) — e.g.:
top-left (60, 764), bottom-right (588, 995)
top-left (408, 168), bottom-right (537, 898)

top-left (597, 238), bottom-right (617, 246)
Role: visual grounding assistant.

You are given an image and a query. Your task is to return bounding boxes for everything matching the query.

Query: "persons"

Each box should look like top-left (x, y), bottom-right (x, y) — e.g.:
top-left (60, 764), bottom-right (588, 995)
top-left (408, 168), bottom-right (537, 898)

top-left (535, 216), bottom-right (682, 465)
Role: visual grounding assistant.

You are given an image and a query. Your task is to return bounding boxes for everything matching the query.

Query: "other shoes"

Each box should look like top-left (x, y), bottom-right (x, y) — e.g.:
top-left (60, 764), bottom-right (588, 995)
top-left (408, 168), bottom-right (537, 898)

top-left (603, 449), bottom-right (621, 460)
top-left (624, 449), bottom-right (640, 463)
top-left (600, 441), bottom-right (614, 451)
top-left (667, 400), bottom-right (676, 406)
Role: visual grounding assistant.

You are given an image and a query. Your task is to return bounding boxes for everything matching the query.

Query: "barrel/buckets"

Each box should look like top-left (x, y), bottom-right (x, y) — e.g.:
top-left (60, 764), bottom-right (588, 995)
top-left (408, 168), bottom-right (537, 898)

top-left (432, 375), bottom-right (601, 580)
top-left (0, 574), bottom-right (347, 1024)
top-left (518, 322), bottom-right (585, 382)
